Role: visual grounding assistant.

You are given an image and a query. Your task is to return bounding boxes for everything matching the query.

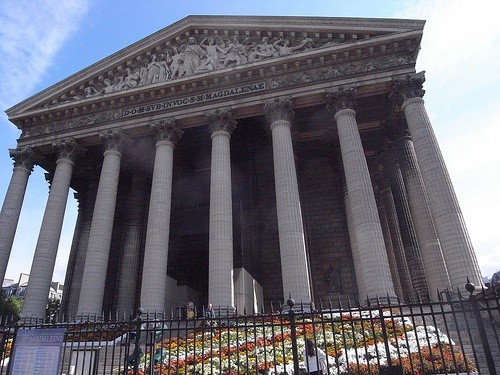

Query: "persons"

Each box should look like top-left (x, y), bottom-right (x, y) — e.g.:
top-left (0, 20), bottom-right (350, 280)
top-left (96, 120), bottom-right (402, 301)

top-left (206, 304), bottom-right (217, 334)
top-left (187, 309), bottom-right (194, 319)
top-left (186, 301), bottom-right (194, 309)
top-left (301, 338), bottom-right (326, 375)
